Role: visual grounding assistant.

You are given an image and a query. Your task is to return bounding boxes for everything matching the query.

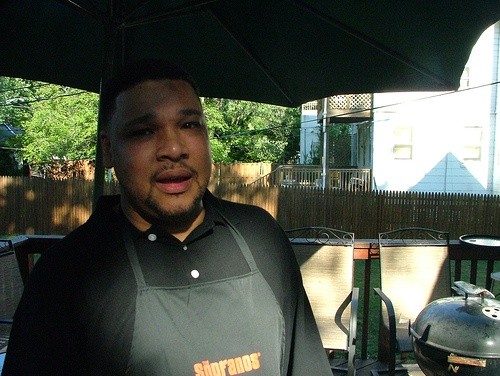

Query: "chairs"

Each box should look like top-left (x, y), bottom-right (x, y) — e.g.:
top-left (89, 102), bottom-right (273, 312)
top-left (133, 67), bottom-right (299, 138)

top-left (284, 227), bottom-right (359, 376)
top-left (374, 228), bottom-right (472, 375)
top-left (0, 239), bottom-right (26, 354)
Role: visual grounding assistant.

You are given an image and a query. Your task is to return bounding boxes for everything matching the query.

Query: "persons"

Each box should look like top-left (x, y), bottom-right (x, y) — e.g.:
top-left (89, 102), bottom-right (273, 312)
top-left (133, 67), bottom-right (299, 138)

top-left (0, 58), bottom-right (335, 376)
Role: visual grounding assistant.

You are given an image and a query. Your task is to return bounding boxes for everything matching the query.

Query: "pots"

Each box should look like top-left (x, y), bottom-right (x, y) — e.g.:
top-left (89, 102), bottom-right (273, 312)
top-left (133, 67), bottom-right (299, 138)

top-left (409, 281), bottom-right (500, 376)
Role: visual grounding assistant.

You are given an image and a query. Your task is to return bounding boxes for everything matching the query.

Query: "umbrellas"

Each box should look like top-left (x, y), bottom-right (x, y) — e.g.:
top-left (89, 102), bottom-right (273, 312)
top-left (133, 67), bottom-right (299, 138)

top-left (0, 0), bottom-right (500, 212)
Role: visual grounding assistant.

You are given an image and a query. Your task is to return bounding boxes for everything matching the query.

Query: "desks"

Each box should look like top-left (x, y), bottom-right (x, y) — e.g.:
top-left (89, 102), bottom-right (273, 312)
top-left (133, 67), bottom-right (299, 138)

top-left (459, 232), bottom-right (500, 293)
